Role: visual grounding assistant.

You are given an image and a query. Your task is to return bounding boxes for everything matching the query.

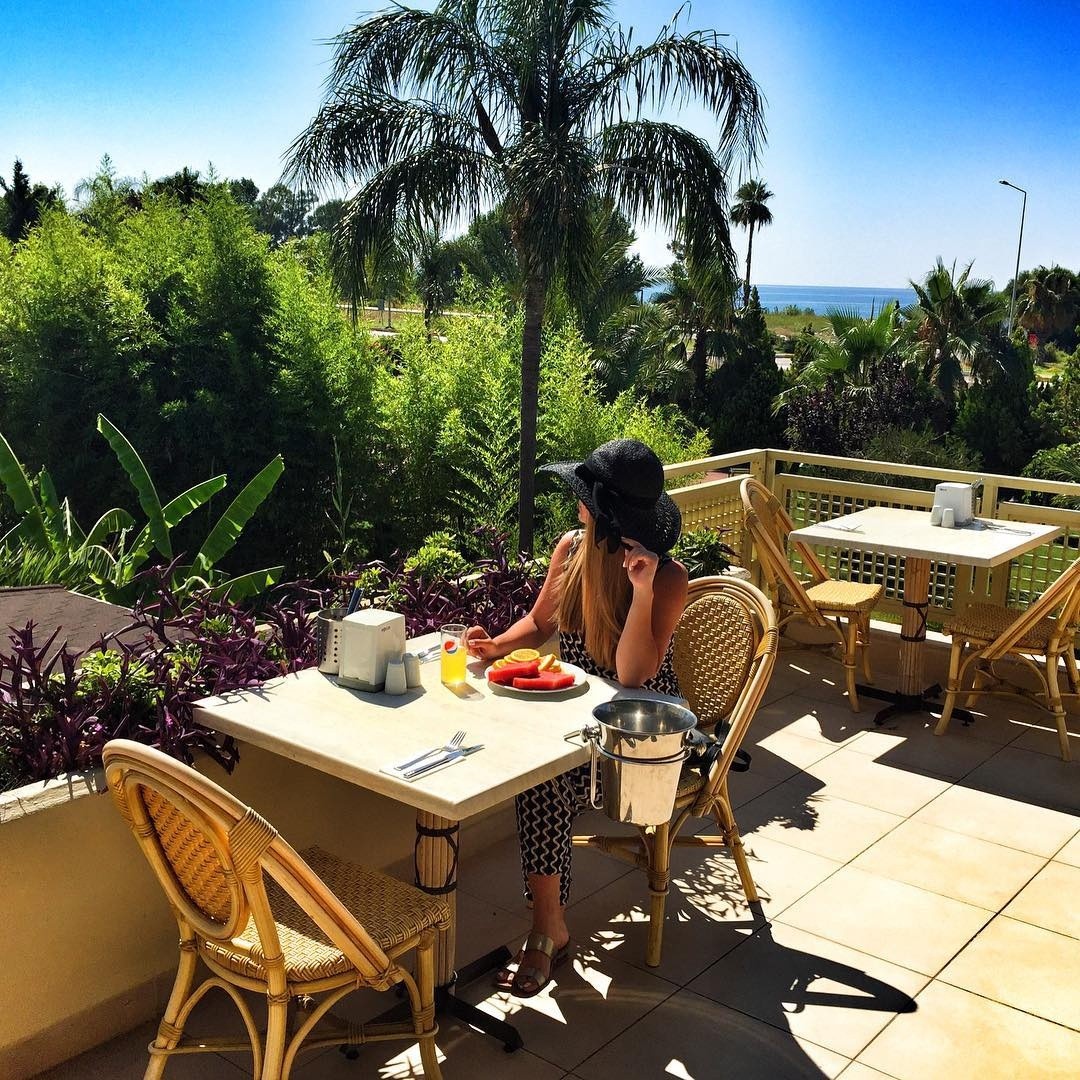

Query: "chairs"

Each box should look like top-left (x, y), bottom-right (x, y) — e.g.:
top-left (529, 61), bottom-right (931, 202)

top-left (524, 575), bottom-right (780, 967)
top-left (932, 557), bottom-right (1080, 762)
top-left (101, 737), bottom-right (452, 1080)
top-left (739, 479), bottom-right (887, 715)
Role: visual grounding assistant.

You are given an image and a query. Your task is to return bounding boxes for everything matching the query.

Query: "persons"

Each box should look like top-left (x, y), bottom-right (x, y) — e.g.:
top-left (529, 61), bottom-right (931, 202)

top-left (459, 439), bottom-right (689, 998)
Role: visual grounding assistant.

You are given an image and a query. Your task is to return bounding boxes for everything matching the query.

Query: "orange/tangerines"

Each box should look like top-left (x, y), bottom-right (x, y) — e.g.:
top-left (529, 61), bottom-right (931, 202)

top-left (492, 648), bottom-right (563, 672)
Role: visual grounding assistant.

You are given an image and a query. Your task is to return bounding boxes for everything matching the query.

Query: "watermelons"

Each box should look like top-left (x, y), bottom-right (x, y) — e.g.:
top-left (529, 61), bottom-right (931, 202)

top-left (489, 661), bottom-right (575, 689)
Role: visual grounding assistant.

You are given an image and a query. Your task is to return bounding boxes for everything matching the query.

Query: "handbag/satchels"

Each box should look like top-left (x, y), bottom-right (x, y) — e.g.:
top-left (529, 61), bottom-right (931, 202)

top-left (684, 719), bottom-right (750, 780)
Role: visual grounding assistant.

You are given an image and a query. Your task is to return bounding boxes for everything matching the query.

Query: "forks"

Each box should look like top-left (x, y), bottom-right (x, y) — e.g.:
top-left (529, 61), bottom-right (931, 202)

top-left (394, 731), bottom-right (466, 771)
top-left (815, 524), bottom-right (862, 532)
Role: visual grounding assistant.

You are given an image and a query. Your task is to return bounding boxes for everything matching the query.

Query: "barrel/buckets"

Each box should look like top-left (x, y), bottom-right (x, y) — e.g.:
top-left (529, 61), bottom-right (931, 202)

top-left (592, 698), bottom-right (697, 827)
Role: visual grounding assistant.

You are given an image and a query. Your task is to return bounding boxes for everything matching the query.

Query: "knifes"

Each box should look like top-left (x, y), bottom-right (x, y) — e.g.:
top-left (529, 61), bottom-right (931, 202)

top-left (404, 745), bottom-right (484, 778)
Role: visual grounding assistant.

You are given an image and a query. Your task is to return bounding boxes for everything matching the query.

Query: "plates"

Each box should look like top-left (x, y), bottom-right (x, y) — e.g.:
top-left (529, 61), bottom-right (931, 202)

top-left (484, 660), bottom-right (587, 693)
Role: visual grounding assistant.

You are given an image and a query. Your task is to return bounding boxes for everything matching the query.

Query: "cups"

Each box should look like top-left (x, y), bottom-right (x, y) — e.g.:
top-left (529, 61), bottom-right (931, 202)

top-left (930, 505), bottom-right (942, 526)
top-left (942, 509), bottom-right (954, 527)
top-left (405, 653), bottom-right (421, 687)
top-left (385, 662), bottom-right (407, 695)
top-left (441, 624), bottom-right (467, 684)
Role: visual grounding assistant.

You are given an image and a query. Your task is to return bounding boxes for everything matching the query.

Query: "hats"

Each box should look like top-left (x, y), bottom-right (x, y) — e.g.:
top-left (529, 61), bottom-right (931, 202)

top-left (540, 438), bottom-right (683, 555)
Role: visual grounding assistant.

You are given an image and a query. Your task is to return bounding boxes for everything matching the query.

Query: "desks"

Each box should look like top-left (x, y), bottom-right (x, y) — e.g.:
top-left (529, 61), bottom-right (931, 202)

top-left (184, 631), bottom-right (690, 1062)
top-left (787, 506), bottom-right (1061, 728)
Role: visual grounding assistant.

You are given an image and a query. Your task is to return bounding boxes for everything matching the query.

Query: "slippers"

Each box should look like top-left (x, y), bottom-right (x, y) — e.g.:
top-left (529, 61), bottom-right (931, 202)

top-left (510, 932), bottom-right (574, 998)
top-left (491, 939), bottom-right (530, 989)
